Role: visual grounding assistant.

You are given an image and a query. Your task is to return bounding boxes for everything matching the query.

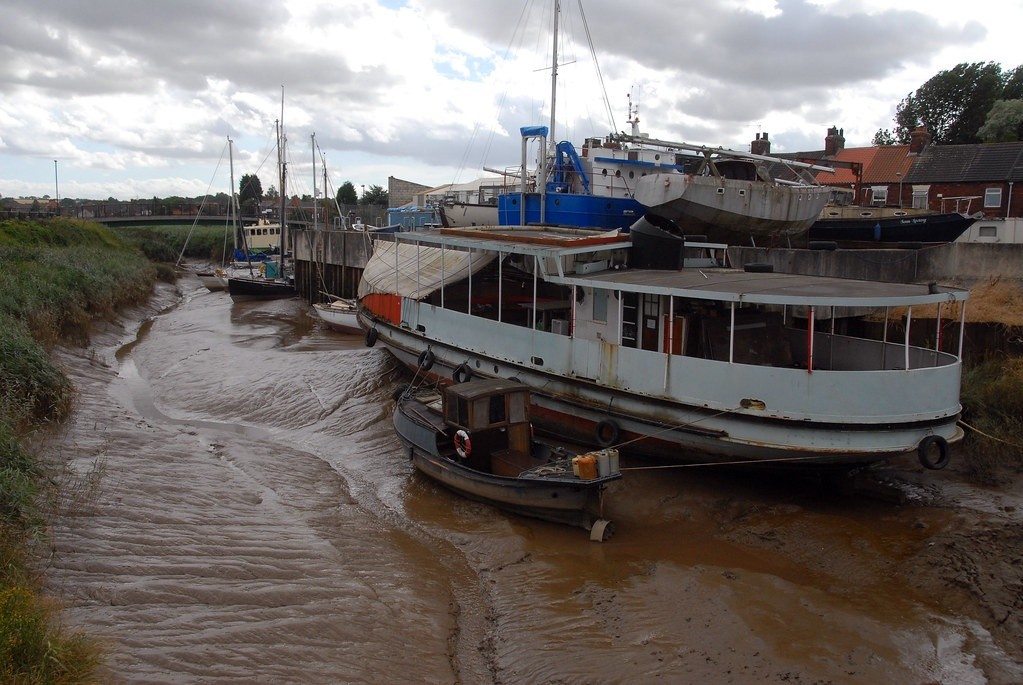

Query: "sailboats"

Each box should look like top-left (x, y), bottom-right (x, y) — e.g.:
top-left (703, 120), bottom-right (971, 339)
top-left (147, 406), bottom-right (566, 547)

top-left (173, 84), bottom-right (350, 303)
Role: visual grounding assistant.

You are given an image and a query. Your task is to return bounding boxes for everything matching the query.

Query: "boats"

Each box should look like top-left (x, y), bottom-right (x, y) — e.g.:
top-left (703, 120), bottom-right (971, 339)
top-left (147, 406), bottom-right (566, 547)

top-left (312, 290), bottom-right (367, 338)
top-left (351, 223), bottom-right (401, 233)
top-left (354, 211), bottom-right (966, 490)
top-left (392, 375), bottom-right (624, 535)
top-left (428, 0), bottom-right (980, 247)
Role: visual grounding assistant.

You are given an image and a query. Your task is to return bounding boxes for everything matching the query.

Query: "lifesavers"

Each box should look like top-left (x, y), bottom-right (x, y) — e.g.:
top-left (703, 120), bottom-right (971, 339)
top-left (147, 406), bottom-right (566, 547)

top-left (454, 430), bottom-right (470, 457)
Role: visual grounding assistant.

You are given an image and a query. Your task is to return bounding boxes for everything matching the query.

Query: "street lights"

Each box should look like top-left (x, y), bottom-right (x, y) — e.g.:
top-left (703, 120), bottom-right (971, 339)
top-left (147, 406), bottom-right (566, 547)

top-left (1006, 181), bottom-right (1014, 218)
top-left (360, 185), bottom-right (365, 203)
top-left (895, 172), bottom-right (903, 204)
top-left (54, 160), bottom-right (59, 215)
